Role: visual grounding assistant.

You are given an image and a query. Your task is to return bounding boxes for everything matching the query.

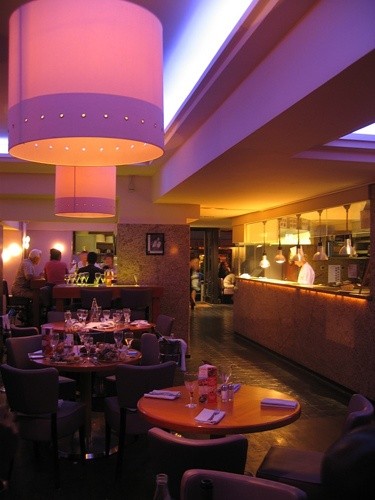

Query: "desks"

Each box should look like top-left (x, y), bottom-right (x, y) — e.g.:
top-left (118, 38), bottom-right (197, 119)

top-left (31, 279), bottom-right (48, 334)
top-left (41, 321), bottom-right (156, 344)
top-left (29, 345), bottom-right (143, 464)
top-left (137, 384), bottom-right (301, 438)
top-left (54, 283), bottom-right (163, 325)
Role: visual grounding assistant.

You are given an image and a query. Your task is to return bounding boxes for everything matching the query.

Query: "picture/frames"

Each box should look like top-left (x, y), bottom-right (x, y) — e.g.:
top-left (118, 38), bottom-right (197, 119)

top-left (146, 232), bottom-right (164, 256)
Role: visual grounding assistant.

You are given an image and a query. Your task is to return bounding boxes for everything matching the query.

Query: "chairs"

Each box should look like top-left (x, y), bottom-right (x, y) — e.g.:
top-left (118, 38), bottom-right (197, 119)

top-left (0, 278), bottom-right (375, 500)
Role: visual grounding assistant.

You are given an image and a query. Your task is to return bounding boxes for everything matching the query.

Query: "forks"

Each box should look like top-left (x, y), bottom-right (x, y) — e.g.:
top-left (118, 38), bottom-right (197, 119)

top-left (208, 408), bottom-right (220, 421)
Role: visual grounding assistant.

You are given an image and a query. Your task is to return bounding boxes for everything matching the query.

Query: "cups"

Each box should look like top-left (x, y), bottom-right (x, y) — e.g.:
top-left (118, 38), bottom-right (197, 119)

top-left (96, 341), bottom-right (104, 356)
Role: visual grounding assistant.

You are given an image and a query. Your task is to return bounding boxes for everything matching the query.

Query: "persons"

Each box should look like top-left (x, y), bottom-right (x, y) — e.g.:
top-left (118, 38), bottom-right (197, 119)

top-left (189, 255), bottom-right (234, 309)
top-left (103, 253), bottom-right (113, 274)
top-left (70, 251), bottom-right (100, 274)
top-left (11, 249), bottom-right (43, 326)
top-left (44, 248), bottom-right (69, 307)
top-left (77, 252), bottom-right (104, 284)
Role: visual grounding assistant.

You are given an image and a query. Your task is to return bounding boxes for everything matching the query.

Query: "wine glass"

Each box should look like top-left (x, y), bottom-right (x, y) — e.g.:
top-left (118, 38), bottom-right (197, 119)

top-left (184, 371), bottom-right (199, 409)
top-left (218, 365), bottom-right (232, 389)
top-left (103, 310), bottom-right (111, 322)
top-left (77, 308), bottom-right (88, 327)
top-left (113, 330), bottom-right (123, 349)
top-left (112, 307), bottom-right (131, 329)
top-left (48, 333), bottom-right (60, 358)
top-left (78, 330), bottom-right (90, 347)
top-left (134, 274), bottom-right (139, 286)
top-left (63, 272), bottom-right (89, 287)
top-left (83, 336), bottom-right (94, 360)
top-left (94, 273), bottom-right (104, 285)
top-left (124, 331), bottom-right (134, 350)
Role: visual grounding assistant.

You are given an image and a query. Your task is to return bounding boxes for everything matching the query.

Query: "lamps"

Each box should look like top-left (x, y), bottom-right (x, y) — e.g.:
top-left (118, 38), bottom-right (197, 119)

top-left (339, 203), bottom-right (357, 255)
top-left (312, 209), bottom-right (328, 261)
top-left (55, 165), bottom-right (117, 218)
top-left (291, 214), bottom-right (305, 261)
top-left (275, 218), bottom-right (286, 264)
top-left (8, 0), bottom-right (164, 166)
top-left (259, 221), bottom-right (271, 268)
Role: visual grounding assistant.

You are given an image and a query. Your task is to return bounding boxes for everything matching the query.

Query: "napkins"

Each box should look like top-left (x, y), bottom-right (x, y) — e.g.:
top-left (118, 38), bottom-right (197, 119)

top-left (195, 408), bottom-right (226, 424)
top-left (144, 390), bottom-right (181, 401)
top-left (218, 384), bottom-right (241, 393)
top-left (260, 398), bottom-right (298, 409)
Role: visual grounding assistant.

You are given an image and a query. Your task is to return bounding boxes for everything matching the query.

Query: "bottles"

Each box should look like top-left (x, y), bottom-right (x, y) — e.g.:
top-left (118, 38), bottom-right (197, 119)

top-left (103, 269), bottom-right (113, 286)
top-left (228, 384), bottom-right (233, 402)
top-left (64, 310), bottom-right (71, 327)
top-left (90, 297), bottom-right (104, 323)
top-left (221, 384), bottom-right (228, 402)
